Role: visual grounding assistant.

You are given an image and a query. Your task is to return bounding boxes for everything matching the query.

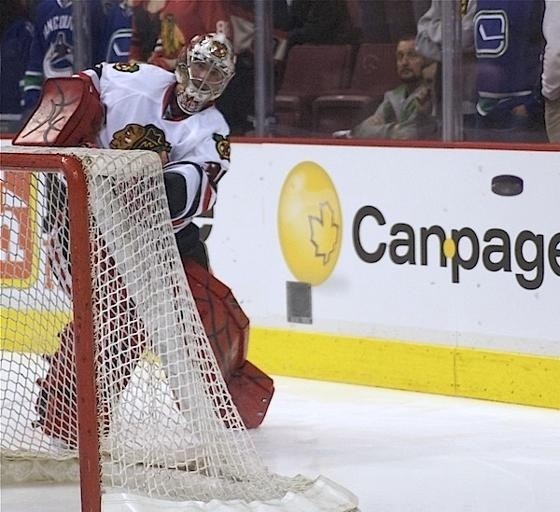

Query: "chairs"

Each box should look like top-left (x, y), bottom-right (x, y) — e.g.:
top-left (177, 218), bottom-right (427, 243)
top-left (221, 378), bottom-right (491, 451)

top-left (273, 42), bottom-right (405, 137)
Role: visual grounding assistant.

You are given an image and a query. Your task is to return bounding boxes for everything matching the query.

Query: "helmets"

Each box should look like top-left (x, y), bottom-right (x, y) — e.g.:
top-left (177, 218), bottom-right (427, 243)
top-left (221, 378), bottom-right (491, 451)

top-left (174, 30), bottom-right (239, 102)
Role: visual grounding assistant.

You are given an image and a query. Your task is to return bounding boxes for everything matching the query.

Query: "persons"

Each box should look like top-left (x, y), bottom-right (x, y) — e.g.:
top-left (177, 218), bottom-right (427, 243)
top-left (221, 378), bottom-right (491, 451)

top-left (1, 2), bottom-right (291, 142)
top-left (10, 31), bottom-right (276, 453)
top-left (415, 2), bottom-right (478, 130)
top-left (350, 33), bottom-right (437, 141)
top-left (473, 2), bottom-right (547, 131)
top-left (543, 2), bottom-right (560, 143)
